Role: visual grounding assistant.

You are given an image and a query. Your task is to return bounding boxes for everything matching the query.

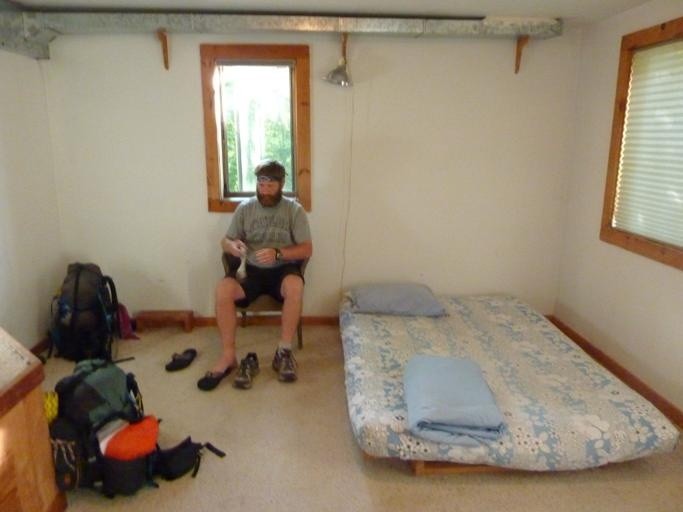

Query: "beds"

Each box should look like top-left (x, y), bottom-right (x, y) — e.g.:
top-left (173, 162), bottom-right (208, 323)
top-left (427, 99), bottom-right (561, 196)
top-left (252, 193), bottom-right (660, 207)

top-left (338, 285), bottom-right (681, 477)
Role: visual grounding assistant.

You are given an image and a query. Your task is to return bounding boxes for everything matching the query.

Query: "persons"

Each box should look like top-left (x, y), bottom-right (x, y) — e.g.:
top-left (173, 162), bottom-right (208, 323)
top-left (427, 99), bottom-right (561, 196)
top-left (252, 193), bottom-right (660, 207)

top-left (194, 157), bottom-right (315, 390)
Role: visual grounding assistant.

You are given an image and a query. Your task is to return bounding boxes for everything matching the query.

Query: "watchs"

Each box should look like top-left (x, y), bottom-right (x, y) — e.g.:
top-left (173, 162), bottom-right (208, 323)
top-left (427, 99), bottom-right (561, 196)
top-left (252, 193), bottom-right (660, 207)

top-left (272, 247), bottom-right (284, 262)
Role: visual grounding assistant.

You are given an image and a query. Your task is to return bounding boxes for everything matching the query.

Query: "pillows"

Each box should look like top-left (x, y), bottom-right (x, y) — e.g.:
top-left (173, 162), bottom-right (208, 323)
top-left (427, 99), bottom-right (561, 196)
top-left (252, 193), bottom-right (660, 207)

top-left (352, 281), bottom-right (445, 316)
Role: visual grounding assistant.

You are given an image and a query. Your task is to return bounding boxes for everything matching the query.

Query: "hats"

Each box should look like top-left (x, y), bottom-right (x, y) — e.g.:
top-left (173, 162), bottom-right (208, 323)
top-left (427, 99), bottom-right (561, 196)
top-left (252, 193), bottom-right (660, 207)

top-left (255, 162), bottom-right (285, 179)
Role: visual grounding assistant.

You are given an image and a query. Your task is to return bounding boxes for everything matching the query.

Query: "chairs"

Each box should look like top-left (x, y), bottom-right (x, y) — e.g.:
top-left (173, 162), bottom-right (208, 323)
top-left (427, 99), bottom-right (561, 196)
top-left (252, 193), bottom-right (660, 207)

top-left (217, 252), bottom-right (308, 350)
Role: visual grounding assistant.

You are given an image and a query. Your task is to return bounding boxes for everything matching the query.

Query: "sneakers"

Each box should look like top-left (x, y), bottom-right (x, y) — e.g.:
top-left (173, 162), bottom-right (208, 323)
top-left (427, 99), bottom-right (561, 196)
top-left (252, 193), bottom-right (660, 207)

top-left (232, 353), bottom-right (259, 389)
top-left (272, 350), bottom-right (297, 381)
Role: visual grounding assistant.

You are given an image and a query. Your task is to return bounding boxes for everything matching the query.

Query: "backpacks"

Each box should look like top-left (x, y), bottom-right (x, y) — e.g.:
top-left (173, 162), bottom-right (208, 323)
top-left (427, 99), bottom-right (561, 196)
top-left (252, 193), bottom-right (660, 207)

top-left (51, 264), bottom-right (119, 359)
top-left (42, 360), bottom-right (201, 495)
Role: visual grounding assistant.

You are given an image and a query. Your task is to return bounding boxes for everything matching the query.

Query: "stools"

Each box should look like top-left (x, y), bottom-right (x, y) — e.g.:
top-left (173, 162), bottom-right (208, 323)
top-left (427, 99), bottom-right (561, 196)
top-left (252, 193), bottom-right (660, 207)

top-left (135, 311), bottom-right (194, 333)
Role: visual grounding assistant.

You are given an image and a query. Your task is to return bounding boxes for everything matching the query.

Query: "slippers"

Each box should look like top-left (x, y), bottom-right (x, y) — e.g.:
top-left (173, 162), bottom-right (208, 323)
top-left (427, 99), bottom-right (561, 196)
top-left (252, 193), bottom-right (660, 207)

top-left (198, 367), bottom-right (229, 390)
top-left (166, 349), bottom-right (196, 371)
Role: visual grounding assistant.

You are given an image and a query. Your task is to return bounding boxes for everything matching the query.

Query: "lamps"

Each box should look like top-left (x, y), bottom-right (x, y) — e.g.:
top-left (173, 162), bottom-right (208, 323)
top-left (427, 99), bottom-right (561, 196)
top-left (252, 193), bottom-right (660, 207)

top-left (323, 30), bottom-right (352, 91)
